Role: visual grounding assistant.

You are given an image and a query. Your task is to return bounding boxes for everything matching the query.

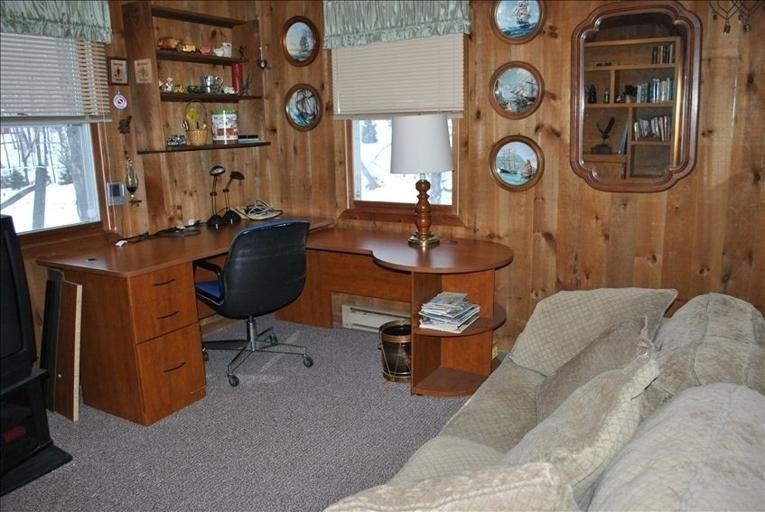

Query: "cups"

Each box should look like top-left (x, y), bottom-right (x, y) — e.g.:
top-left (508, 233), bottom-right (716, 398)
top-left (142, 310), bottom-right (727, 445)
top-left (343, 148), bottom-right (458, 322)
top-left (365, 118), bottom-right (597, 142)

top-left (201, 75), bottom-right (214, 86)
top-left (202, 86), bottom-right (211, 95)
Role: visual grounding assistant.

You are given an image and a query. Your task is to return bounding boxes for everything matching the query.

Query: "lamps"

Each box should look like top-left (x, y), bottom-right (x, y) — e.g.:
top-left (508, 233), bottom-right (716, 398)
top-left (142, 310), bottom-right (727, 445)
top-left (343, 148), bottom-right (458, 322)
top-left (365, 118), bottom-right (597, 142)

top-left (222, 170), bottom-right (245, 224)
top-left (392, 115), bottom-right (454, 246)
top-left (208, 166), bottom-right (225, 228)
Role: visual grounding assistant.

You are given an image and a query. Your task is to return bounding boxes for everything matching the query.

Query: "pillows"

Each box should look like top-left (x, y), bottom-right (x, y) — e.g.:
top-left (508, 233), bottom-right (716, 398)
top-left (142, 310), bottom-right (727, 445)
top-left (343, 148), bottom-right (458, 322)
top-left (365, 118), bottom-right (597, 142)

top-left (508, 288), bottom-right (680, 378)
top-left (535, 314), bottom-right (658, 424)
top-left (503, 366), bottom-right (642, 497)
top-left (325, 463), bottom-right (583, 512)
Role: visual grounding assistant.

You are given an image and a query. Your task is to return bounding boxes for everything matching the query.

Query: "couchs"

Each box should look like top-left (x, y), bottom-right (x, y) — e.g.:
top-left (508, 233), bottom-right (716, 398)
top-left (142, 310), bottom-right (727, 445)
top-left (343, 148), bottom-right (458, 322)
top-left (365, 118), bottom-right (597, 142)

top-left (326, 292), bottom-right (765, 511)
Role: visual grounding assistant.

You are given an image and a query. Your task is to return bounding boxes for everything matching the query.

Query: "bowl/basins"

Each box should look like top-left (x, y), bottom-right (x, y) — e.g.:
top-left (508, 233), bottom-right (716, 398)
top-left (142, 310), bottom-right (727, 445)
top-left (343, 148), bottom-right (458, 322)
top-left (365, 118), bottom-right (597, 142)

top-left (187, 86), bottom-right (205, 95)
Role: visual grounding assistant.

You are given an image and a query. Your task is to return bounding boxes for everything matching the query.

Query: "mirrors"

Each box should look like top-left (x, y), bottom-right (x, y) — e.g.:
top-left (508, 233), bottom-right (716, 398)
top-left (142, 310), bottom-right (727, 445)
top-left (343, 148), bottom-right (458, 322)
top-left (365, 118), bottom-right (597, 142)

top-left (570, 0), bottom-right (702, 193)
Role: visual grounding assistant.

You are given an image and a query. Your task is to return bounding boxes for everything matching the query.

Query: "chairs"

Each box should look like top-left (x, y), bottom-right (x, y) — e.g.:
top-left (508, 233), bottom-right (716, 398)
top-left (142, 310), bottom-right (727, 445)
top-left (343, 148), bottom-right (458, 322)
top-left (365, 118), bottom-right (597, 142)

top-left (193, 220), bottom-right (314, 386)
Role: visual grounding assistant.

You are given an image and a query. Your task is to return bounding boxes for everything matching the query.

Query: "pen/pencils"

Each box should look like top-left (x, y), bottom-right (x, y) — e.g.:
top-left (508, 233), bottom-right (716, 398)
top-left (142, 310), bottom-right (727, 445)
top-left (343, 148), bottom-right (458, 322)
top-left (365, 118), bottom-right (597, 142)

top-left (196, 121), bottom-right (200, 129)
top-left (184, 119), bottom-right (190, 130)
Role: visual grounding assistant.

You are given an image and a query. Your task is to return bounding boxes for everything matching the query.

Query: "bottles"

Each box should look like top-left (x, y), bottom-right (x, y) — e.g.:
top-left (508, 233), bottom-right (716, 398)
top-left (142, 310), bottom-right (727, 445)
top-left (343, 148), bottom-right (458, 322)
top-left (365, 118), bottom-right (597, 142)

top-left (587, 84), bottom-right (597, 103)
top-left (603, 88), bottom-right (609, 103)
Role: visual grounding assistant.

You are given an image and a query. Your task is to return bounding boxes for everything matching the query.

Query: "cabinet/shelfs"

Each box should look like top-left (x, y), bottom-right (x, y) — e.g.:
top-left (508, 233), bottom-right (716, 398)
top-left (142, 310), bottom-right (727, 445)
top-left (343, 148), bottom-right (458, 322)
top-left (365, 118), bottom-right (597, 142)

top-left (582, 23), bottom-right (684, 179)
top-left (123, 2), bottom-right (272, 154)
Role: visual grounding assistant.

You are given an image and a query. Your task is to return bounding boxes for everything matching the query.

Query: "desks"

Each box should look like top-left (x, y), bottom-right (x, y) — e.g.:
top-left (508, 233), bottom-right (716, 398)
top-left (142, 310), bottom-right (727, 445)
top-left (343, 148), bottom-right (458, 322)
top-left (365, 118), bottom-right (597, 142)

top-left (37, 218), bottom-right (334, 427)
top-left (276, 227), bottom-right (513, 399)
top-left (0, 366), bottom-right (73, 499)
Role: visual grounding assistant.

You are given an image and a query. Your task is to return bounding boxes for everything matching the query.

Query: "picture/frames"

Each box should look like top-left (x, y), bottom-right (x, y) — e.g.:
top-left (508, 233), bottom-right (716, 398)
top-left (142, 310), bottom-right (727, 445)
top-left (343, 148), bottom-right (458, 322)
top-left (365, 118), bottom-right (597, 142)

top-left (488, 61), bottom-right (544, 119)
top-left (489, 135), bottom-right (545, 192)
top-left (279, 16), bottom-right (320, 66)
top-left (489, 0), bottom-right (547, 45)
top-left (108, 56), bottom-right (130, 86)
top-left (283, 85), bottom-right (322, 132)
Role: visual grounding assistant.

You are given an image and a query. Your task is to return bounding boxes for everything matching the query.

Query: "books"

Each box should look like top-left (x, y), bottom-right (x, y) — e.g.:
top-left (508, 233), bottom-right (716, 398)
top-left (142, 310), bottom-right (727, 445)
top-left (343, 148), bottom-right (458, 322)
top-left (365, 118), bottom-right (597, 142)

top-left (617, 121), bottom-right (628, 154)
top-left (633, 76), bottom-right (672, 103)
top-left (651, 42), bottom-right (674, 64)
top-left (633, 114), bottom-right (671, 142)
top-left (417, 289), bottom-right (483, 334)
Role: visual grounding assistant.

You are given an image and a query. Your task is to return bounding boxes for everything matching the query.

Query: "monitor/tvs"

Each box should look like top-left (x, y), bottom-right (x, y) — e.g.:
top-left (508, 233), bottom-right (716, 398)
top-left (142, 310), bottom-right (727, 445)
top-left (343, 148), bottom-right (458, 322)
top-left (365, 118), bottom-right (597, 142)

top-left (1, 213), bottom-right (37, 392)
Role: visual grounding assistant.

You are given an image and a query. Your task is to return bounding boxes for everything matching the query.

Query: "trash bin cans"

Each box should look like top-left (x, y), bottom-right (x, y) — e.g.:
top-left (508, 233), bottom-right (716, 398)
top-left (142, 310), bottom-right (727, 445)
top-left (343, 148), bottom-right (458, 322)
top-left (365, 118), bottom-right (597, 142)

top-left (378, 320), bottom-right (412, 383)
